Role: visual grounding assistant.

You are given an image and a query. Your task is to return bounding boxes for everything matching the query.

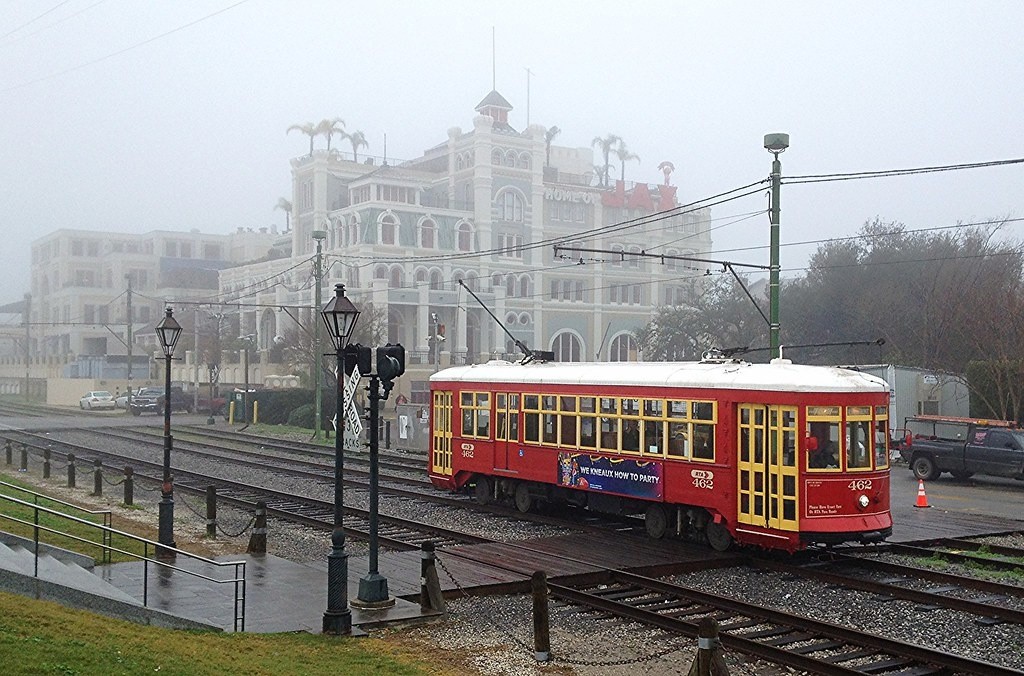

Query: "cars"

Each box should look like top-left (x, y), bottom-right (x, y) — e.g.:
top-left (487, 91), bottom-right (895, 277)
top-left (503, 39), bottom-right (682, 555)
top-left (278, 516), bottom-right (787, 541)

top-left (79, 389), bottom-right (137, 409)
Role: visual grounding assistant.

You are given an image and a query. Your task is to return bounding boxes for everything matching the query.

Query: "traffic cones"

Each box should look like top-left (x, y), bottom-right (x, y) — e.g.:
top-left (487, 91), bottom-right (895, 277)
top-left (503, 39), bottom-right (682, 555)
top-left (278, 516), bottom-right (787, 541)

top-left (913, 478), bottom-right (931, 508)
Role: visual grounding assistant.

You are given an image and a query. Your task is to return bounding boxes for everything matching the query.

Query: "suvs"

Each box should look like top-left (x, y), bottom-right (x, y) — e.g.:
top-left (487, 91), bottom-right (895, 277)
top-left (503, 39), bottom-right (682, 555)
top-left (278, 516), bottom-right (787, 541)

top-left (132, 385), bottom-right (195, 416)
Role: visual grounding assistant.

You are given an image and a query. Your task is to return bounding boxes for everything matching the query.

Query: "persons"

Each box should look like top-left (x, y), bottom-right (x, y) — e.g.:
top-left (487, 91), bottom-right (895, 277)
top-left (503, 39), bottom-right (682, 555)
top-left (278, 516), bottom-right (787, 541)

top-left (819, 441), bottom-right (839, 467)
top-left (645, 421), bottom-right (662, 437)
top-left (623, 420), bottom-right (640, 451)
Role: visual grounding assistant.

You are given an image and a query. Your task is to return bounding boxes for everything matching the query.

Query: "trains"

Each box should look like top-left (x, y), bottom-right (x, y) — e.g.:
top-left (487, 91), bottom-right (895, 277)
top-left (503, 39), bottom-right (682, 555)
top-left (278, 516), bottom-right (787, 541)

top-left (427, 280), bottom-right (893, 551)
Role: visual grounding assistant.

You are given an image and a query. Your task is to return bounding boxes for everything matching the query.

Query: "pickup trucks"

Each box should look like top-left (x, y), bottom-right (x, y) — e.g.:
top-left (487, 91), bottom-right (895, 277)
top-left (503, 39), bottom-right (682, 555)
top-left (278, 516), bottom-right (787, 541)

top-left (900, 424), bottom-right (1024, 482)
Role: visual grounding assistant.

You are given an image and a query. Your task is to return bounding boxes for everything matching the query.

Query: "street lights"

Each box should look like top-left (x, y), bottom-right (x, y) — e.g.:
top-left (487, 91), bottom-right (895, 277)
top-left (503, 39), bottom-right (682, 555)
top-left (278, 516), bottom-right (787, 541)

top-left (154, 305), bottom-right (183, 560)
top-left (319, 284), bottom-right (361, 634)
